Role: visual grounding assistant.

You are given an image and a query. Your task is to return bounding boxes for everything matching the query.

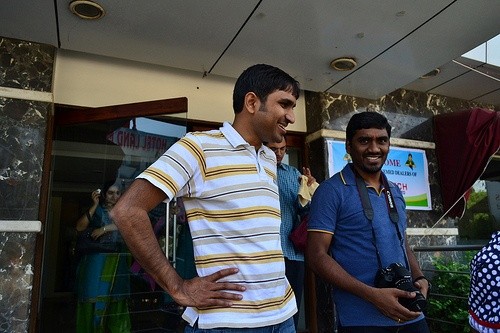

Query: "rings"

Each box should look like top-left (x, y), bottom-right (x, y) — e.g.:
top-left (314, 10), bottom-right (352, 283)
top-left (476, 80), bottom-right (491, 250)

top-left (397, 318), bottom-right (401, 323)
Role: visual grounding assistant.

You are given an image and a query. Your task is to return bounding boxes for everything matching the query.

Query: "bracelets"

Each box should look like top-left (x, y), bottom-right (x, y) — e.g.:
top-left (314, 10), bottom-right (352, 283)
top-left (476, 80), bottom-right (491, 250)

top-left (104, 224), bottom-right (108, 234)
top-left (88, 209), bottom-right (93, 218)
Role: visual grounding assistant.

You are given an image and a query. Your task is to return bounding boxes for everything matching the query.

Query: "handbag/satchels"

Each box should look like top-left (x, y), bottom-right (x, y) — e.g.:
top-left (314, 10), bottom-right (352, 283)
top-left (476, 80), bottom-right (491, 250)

top-left (74, 211), bottom-right (118, 254)
top-left (288, 215), bottom-right (309, 252)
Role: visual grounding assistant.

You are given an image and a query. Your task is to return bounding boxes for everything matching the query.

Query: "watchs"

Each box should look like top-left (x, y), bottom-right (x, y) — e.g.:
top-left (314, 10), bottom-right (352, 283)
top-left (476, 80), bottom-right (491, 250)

top-left (412, 275), bottom-right (433, 292)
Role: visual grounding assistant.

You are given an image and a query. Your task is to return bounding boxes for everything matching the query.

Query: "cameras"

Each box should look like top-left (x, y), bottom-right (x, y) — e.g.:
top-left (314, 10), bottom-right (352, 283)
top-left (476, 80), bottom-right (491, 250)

top-left (375, 263), bottom-right (427, 312)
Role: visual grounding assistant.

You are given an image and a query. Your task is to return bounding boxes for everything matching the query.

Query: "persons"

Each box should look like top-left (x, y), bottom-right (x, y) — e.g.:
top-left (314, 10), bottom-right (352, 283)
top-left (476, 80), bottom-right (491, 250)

top-left (68, 178), bottom-right (133, 333)
top-left (106, 63), bottom-right (302, 333)
top-left (467, 231), bottom-right (500, 333)
top-left (306, 110), bottom-right (433, 333)
top-left (262, 132), bottom-right (317, 332)
